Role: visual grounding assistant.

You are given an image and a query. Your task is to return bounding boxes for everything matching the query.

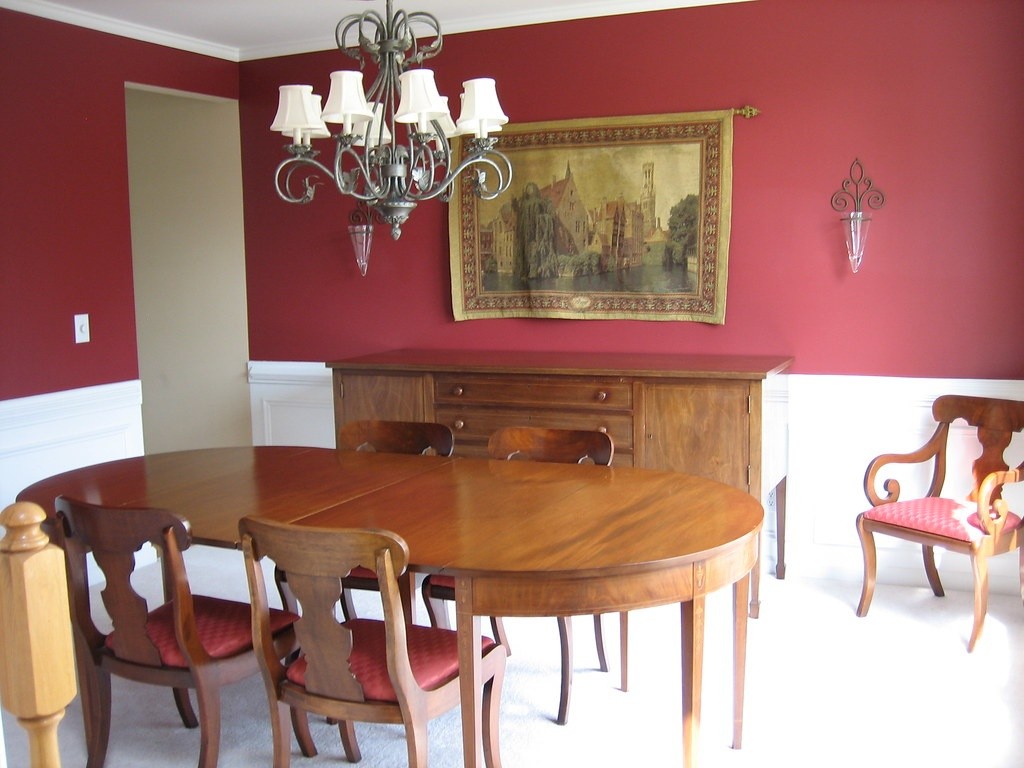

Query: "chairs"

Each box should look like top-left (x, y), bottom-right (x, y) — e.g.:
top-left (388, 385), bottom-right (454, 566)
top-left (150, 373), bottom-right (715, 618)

top-left (422, 424), bottom-right (614, 726)
top-left (54, 496), bottom-right (319, 768)
top-left (856, 395), bottom-right (1024, 654)
top-left (273, 419), bottom-right (454, 724)
top-left (239, 517), bottom-right (507, 768)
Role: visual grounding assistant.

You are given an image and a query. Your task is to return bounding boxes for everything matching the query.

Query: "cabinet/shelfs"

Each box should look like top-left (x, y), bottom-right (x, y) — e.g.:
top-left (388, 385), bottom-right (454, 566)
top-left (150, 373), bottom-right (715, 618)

top-left (325, 350), bottom-right (796, 619)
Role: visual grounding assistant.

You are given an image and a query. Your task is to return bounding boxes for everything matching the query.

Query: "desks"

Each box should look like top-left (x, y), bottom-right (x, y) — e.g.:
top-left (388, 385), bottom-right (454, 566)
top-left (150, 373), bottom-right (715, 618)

top-left (14, 445), bottom-right (764, 768)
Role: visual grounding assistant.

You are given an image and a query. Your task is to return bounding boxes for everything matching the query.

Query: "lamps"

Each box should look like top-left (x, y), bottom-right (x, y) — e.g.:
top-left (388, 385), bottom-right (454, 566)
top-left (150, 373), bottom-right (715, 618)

top-left (831, 159), bottom-right (886, 273)
top-left (348, 197), bottom-right (387, 276)
top-left (270, 0), bottom-right (513, 240)
